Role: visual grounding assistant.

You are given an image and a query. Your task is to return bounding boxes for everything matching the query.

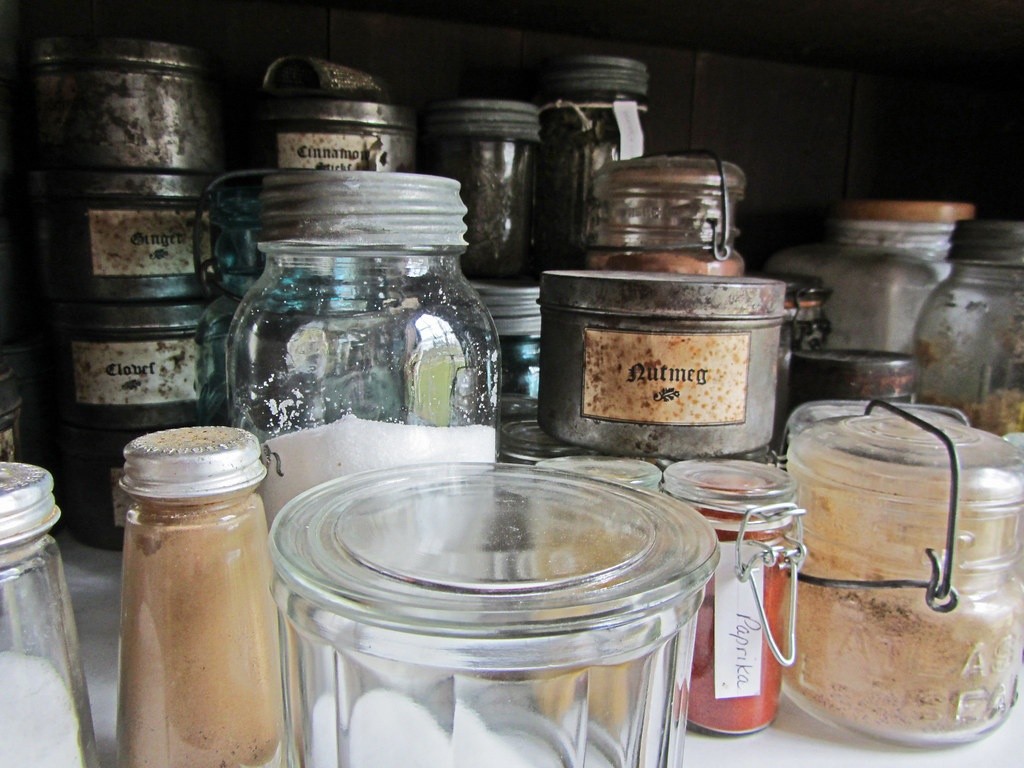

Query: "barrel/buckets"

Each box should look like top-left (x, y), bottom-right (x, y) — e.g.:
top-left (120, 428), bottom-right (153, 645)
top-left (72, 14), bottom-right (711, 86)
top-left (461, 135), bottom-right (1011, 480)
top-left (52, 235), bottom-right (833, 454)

top-left (0, 18), bottom-right (222, 552)
top-left (540, 258), bottom-right (783, 469)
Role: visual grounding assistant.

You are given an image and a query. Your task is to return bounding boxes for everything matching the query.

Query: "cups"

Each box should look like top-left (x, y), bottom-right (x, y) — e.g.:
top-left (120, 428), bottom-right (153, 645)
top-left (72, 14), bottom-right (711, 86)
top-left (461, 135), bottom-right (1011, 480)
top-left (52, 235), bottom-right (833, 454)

top-left (783, 399), bottom-right (1023, 749)
top-left (261, 463), bottom-right (722, 768)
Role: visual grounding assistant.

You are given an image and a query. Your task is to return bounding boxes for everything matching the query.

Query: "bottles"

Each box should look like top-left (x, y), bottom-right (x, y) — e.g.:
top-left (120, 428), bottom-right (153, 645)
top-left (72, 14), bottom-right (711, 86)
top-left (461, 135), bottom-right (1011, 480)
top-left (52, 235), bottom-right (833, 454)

top-left (667, 459), bottom-right (808, 735)
top-left (264, 98), bottom-right (418, 171)
top-left (428, 97), bottom-right (536, 286)
top-left (470, 283), bottom-right (663, 732)
top-left (1, 462), bottom-right (99, 768)
top-left (222, 172), bottom-right (505, 766)
top-left (112, 425), bottom-right (284, 767)
top-left (911, 216), bottom-right (1024, 440)
top-left (743, 199), bottom-right (977, 472)
top-left (534, 50), bottom-right (652, 269)
top-left (589, 152), bottom-right (756, 279)
